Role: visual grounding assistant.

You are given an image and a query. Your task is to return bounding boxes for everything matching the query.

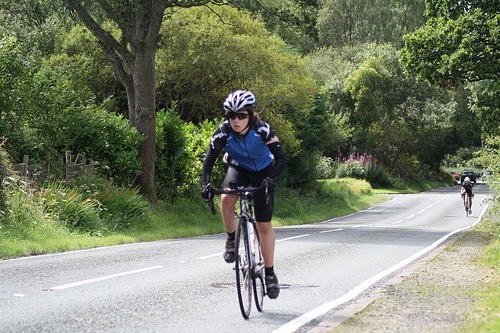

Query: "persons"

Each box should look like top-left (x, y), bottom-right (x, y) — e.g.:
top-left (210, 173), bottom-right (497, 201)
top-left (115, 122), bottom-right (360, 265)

top-left (201, 90), bottom-right (285, 299)
top-left (461, 177), bottom-right (474, 214)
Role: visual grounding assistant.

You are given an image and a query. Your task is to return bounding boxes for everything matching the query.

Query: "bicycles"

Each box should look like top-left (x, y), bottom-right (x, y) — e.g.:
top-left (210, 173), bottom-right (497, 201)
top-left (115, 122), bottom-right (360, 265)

top-left (460, 190), bottom-right (474, 218)
top-left (201, 177), bottom-right (267, 320)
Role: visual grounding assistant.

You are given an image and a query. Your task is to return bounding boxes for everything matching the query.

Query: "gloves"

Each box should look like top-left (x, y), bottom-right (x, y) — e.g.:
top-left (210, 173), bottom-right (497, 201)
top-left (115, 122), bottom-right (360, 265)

top-left (200, 184), bottom-right (211, 199)
top-left (263, 176), bottom-right (276, 192)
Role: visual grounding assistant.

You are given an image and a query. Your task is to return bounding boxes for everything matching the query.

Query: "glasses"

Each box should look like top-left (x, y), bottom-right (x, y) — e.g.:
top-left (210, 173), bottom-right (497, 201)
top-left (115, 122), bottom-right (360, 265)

top-left (228, 113), bottom-right (251, 120)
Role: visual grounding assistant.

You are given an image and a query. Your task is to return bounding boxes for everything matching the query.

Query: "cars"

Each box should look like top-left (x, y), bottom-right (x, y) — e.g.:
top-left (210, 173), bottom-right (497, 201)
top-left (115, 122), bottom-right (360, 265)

top-left (448, 172), bottom-right (460, 181)
top-left (481, 172), bottom-right (493, 181)
top-left (460, 170), bottom-right (477, 184)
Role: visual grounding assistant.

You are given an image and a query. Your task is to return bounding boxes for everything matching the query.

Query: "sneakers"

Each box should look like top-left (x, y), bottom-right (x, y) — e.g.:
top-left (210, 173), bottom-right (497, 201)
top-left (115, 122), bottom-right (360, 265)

top-left (223, 237), bottom-right (236, 263)
top-left (265, 273), bottom-right (280, 299)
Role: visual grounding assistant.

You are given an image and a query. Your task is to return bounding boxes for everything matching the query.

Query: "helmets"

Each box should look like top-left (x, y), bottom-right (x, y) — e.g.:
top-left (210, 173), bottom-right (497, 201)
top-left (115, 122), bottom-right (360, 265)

top-left (223, 89), bottom-right (255, 114)
